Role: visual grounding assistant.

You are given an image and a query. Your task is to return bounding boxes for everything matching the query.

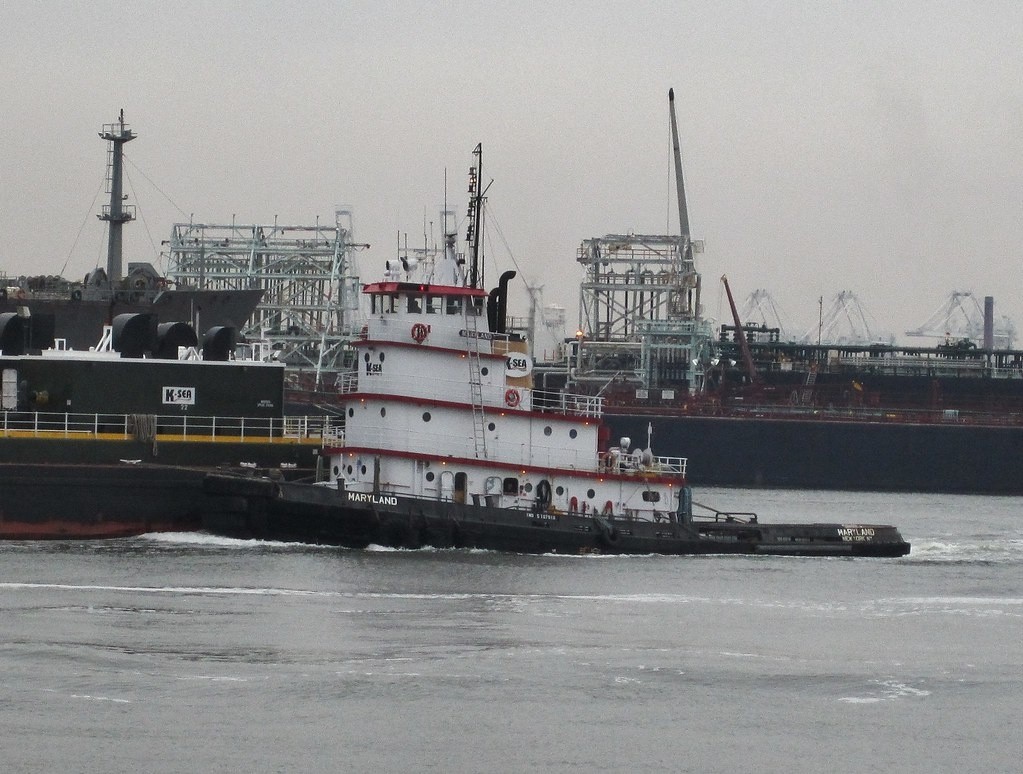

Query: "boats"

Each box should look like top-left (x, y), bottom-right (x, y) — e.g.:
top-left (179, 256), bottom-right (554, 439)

top-left (0, 108), bottom-right (347, 540)
top-left (200, 142), bottom-right (912, 559)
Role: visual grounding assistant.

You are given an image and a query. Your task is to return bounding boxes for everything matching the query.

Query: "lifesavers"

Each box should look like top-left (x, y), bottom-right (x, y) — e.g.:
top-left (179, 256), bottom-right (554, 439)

top-left (505, 389), bottom-right (520, 407)
top-left (361, 327), bottom-right (368, 341)
top-left (602, 526), bottom-right (620, 546)
top-left (601, 453), bottom-right (616, 470)
top-left (411, 323), bottom-right (427, 342)
